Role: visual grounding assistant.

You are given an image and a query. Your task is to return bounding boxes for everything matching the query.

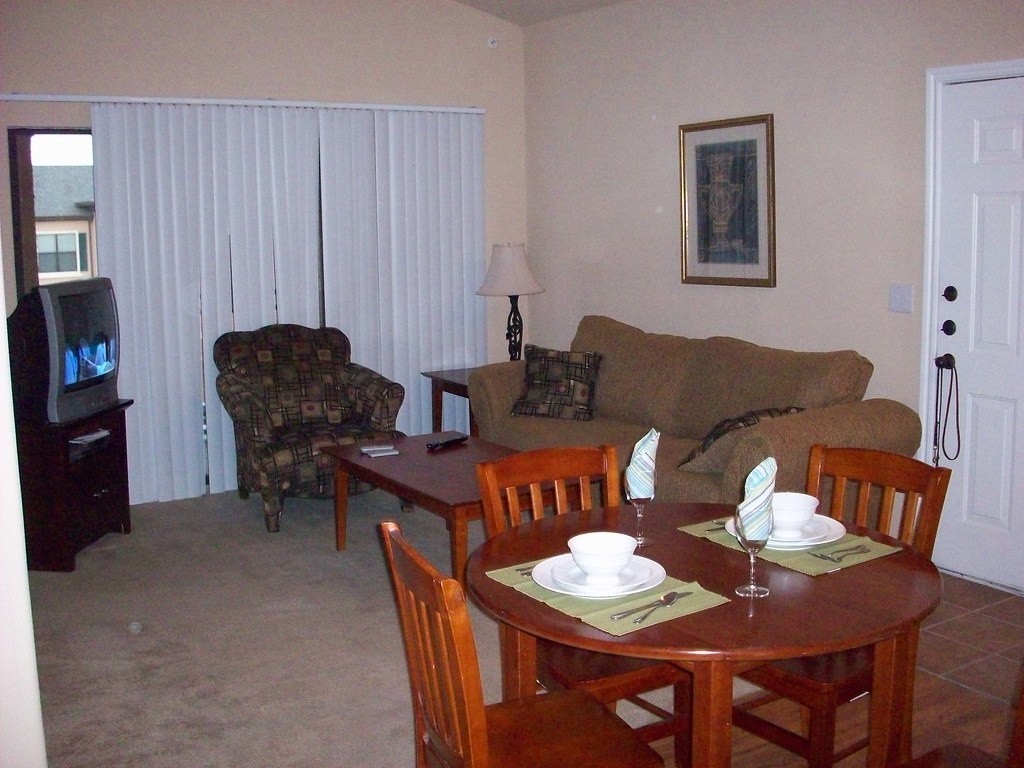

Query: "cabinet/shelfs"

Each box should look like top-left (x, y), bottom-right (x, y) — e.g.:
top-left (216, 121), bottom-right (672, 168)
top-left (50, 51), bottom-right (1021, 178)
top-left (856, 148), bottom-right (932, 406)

top-left (17, 399), bottom-right (135, 572)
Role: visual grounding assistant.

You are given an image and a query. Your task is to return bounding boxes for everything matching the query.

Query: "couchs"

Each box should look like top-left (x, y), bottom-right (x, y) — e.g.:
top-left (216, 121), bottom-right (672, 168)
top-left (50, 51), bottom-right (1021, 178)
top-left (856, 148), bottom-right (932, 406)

top-left (210, 324), bottom-right (413, 533)
top-left (468, 315), bottom-right (923, 534)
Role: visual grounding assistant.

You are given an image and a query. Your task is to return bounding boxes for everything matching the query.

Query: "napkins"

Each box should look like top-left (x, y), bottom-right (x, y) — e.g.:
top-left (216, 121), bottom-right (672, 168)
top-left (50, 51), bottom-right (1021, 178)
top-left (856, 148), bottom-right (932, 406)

top-left (737, 456), bottom-right (778, 541)
top-left (626, 427), bottom-right (660, 501)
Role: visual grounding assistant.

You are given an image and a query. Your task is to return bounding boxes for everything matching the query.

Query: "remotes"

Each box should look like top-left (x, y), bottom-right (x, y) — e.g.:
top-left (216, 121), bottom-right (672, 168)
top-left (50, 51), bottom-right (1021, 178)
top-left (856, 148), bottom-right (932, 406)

top-left (361, 444), bottom-right (400, 458)
top-left (426, 435), bottom-right (468, 448)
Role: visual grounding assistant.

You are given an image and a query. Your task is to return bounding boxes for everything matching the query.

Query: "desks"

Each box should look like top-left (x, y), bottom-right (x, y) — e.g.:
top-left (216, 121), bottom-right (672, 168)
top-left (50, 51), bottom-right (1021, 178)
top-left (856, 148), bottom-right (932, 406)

top-left (466, 501), bottom-right (941, 768)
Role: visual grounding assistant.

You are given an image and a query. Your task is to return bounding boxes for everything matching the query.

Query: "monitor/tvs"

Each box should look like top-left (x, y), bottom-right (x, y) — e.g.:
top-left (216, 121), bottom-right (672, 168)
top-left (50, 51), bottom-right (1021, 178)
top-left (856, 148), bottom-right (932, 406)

top-left (7, 277), bottom-right (120, 424)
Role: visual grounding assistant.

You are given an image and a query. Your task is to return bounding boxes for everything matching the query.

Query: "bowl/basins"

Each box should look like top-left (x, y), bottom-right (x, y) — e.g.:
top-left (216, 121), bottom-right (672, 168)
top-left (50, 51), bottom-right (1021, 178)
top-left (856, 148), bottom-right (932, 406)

top-left (568, 531), bottom-right (638, 581)
top-left (770, 490), bottom-right (820, 539)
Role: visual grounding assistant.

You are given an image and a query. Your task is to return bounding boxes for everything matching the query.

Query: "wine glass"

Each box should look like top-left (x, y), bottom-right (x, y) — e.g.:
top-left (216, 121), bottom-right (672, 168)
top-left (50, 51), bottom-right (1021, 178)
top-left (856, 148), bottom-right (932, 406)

top-left (734, 506), bottom-right (773, 597)
top-left (624, 469), bottom-right (657, 546)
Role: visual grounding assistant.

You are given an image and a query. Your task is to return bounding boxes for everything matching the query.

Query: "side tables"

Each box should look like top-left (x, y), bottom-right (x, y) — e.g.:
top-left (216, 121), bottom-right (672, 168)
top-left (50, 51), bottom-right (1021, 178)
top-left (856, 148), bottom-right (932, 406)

top-left (420, 366), bottom-right (491, 437)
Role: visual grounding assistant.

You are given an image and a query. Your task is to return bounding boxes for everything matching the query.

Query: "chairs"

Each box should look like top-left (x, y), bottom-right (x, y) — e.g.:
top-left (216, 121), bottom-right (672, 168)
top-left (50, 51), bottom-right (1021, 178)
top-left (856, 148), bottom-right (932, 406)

top-left (473, 444), bottom-right (695, 768)
top-left (381, 520), bottom-right (668, 768)
top-left (734, 443), bottom-right (952, 768)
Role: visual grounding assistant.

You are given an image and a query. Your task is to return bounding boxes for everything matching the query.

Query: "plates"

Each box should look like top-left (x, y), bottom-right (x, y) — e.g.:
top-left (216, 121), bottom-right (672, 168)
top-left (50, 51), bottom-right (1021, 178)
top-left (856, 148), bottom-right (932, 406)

top-left (532, 553), bottom-right (667, 599)
top-left (767, 519), bottom-right (831, 542)
top-left (725, 514), bottom-right (847, 551)
top-left (550, 558), bottom-right (653, 592)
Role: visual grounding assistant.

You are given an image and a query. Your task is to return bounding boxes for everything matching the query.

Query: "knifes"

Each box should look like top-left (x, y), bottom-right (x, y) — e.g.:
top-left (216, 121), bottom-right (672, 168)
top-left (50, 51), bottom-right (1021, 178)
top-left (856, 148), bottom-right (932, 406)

top-left (609, 591), bottom-right (693, 620)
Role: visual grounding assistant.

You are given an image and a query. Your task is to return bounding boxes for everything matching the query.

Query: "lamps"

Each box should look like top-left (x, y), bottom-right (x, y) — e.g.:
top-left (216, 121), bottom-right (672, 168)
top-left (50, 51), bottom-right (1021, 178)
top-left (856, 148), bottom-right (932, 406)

top-left (475, 243), bottom-right (545, 362)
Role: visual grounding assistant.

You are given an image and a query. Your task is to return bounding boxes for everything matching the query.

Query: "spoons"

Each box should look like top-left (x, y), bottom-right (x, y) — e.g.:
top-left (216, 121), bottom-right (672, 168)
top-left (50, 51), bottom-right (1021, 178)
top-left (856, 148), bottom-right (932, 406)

top-left (633, 591), bottom-right (678, 625)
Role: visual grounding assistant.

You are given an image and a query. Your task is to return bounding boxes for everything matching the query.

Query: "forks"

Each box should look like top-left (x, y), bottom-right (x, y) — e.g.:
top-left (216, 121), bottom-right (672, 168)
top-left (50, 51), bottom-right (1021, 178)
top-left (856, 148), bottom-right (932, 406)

top-left (807, 545), bottom-right (871, 563)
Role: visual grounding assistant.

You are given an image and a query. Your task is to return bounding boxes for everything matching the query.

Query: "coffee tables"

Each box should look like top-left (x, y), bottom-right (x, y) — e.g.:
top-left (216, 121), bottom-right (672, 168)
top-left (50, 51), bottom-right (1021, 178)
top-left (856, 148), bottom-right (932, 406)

top-left (318, 430), bottom-right (609, 602)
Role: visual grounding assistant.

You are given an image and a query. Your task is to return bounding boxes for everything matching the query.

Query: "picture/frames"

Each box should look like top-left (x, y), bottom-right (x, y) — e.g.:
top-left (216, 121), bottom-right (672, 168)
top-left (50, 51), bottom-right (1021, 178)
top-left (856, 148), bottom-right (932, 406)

top-left (678, 112), bottom-right (776, 287)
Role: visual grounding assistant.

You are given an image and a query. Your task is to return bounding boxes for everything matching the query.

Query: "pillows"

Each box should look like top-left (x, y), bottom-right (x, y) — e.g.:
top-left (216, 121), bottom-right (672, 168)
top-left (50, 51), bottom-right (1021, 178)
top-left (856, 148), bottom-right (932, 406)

top-left (505, 344), bottom-right (602, 422)
top-left (677, 405), bottom-right (805, 468)
